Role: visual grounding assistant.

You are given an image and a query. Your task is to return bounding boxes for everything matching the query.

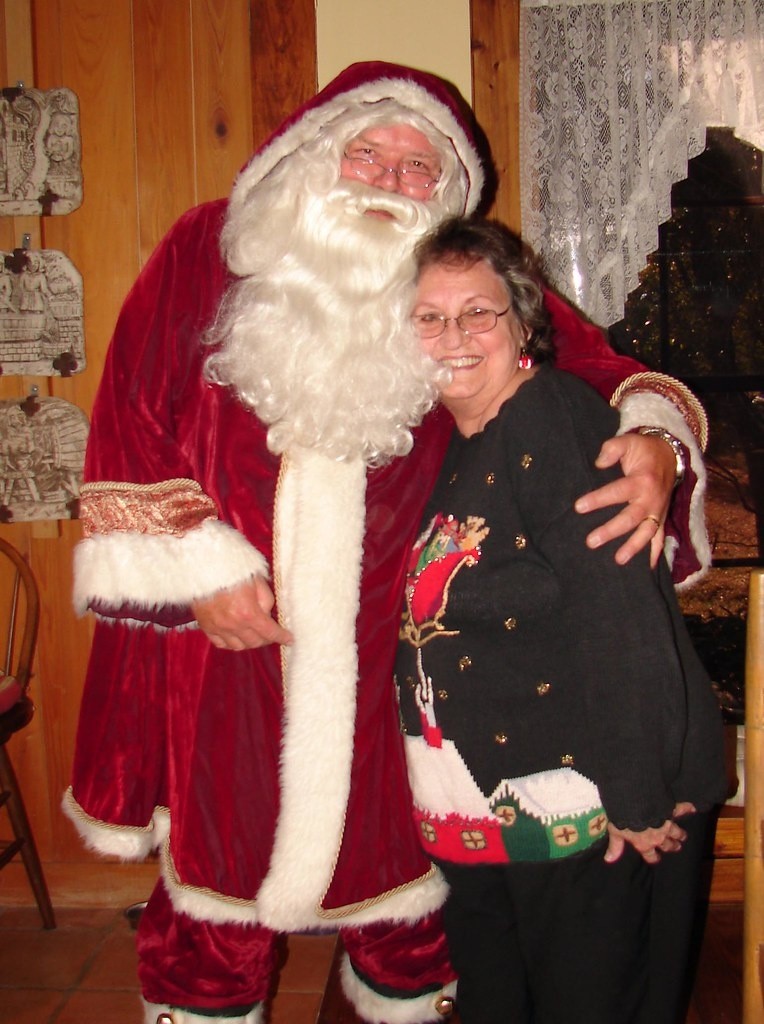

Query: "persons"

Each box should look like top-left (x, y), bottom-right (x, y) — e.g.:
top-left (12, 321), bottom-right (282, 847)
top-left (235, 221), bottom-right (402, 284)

top-left (65, 61), bottom-right (711, 1024)
top-left (393, 217), bottom-right (727, 1024)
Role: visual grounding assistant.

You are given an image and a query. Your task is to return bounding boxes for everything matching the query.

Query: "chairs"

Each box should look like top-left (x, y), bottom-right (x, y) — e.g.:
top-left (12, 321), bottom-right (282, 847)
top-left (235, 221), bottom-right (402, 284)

top-left (0, 538), bottom-right (56, 929)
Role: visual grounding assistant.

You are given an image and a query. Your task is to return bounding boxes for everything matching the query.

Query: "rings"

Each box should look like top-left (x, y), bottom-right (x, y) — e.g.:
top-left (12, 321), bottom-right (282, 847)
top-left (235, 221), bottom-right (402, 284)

top-left (643, 517), bottom-right (659, 529)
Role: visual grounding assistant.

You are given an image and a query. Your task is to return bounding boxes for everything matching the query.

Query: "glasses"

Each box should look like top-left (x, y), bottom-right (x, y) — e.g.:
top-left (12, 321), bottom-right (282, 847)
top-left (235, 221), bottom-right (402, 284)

top-left (407, 305), bottom-right (511, 339)
top-left (342, 149), bottom-right (442, 188)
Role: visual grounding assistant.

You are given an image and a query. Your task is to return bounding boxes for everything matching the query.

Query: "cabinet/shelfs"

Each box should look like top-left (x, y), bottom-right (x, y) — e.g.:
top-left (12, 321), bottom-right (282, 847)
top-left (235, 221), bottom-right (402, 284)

top-left (0, 0), bottom-right (317, 908)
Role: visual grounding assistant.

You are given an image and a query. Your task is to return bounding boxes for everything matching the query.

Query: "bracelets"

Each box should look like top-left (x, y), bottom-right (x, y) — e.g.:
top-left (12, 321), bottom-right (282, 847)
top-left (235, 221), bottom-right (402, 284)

top-left (642, 427), bottom-right (684, 477)
